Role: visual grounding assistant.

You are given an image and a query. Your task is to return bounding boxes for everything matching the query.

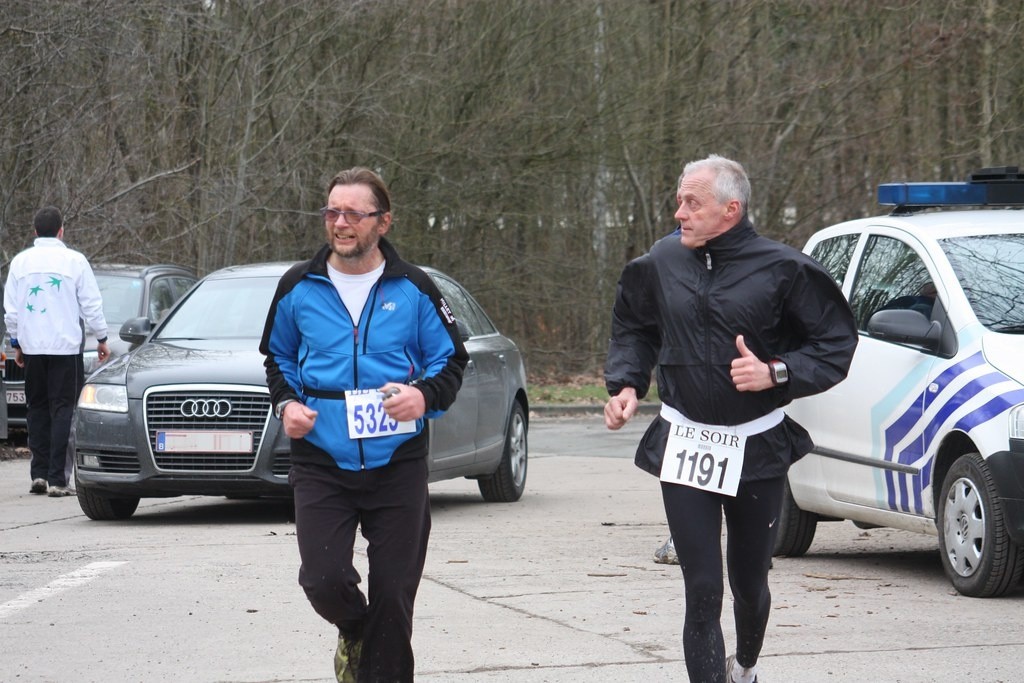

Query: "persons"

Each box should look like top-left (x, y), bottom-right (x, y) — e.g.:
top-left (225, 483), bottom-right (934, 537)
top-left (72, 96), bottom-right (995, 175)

top-left (3, 206), bottom-right (111, 498)
top-left (258, 168), bottom-right (470, 683)
top-left (604, 155), bottom-right (860, 683)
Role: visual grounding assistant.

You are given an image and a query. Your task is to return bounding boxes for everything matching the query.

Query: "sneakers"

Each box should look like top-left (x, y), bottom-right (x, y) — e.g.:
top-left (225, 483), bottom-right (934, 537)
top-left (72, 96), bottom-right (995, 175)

top-left (333, 631), bottom-right (362, 683)
top-left (49, 485), bottom-right (77, 497)
top-left (726, 656), bottom-right (758, 683)
top-left (29, 478), bottom-right (48, 494)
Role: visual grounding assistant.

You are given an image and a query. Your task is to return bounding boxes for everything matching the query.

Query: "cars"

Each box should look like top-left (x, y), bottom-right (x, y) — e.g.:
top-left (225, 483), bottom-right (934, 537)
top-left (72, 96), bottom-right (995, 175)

top-left (770, 169), bottom-right (1023, 601)
top-left (0, 261), bottom-right (200, 429)
top-left (71, 262), bottom-right (530, 522)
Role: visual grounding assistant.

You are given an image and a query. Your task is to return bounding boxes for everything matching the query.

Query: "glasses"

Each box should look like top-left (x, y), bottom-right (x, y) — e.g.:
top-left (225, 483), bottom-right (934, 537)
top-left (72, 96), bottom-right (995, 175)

top-left (320, 206), bottom-right (380, 224)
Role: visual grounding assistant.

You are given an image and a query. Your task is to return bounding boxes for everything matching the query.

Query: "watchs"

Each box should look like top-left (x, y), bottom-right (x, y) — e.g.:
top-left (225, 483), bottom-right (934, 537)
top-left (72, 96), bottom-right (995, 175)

top-left (768, 358), bottom-right (788, 388)
top-left (274, 399), bottom-right (294, 421)
